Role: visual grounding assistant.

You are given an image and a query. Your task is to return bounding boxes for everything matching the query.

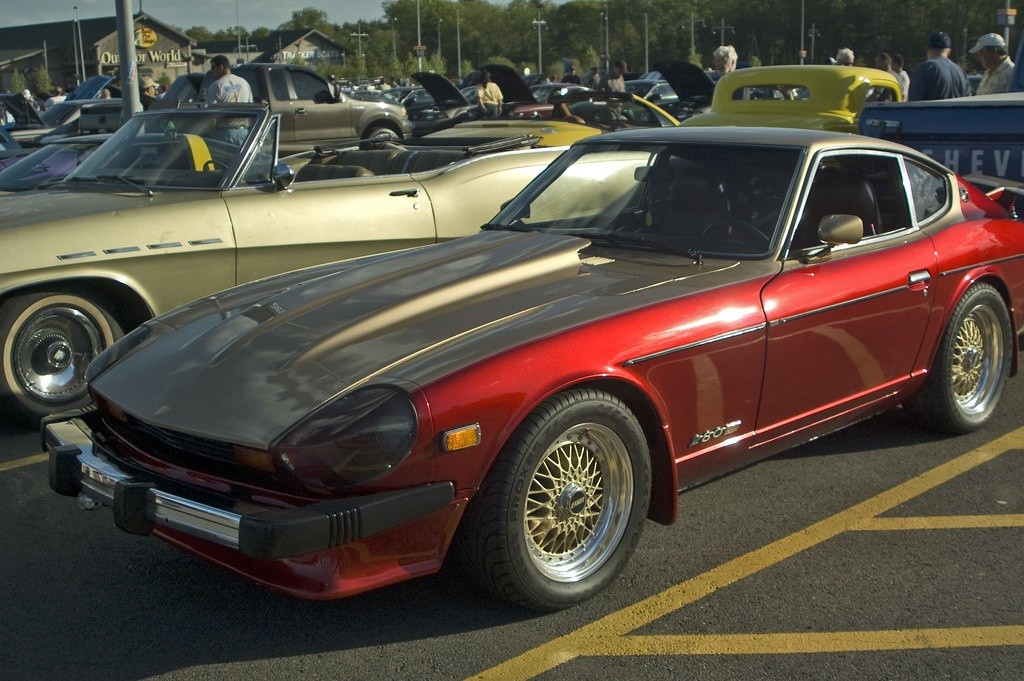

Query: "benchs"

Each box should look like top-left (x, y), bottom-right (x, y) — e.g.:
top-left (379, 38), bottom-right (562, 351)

top-left (323, 151), bottom-right (468, 175)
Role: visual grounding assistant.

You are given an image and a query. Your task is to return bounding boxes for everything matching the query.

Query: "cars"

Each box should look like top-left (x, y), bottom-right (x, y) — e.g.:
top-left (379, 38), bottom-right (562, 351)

top-left (1, 63), bottom-right (242, 199)
top-left (675, 64), bottom-right (902, 135)
top-left (39, 124), bottom-right (1023, 614)
top-left (333, 60), bottom-right (721, 148)
top-left (858, 29), bottom-right (1024, 223)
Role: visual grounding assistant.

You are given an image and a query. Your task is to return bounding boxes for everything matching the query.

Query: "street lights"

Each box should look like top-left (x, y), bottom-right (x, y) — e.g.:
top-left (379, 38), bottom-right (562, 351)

top-left (456, 7), bottom-right (463, 80)
top-left (641, 12), bottom-right (648, 72)
top-left (437, 17), bottom-right (444, 60)
top-left (712, 25), bottom-right (736, 46)
top-left (600, 3), bottom-right (610, 70)
top-left (390, 17), bottom-right (398, 61)
top-left (532, 20), bottom-right (550, 72)
top-left (808, 28), bottom-right (821, 64)
top-left (680, 18), bottom-right (708, 48)
top-left (350, 32), bottom-right (370, 60)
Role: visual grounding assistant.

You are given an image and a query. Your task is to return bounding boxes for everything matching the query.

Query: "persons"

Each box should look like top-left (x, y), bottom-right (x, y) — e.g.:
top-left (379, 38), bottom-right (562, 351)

top-left (367, 78), bottom-right (401, 101)
top-left (835, 47), bottom-right (856, 69)
top-left (869, 52), bottom-right (911, 105)
top-left (475, 69), bottom-right (503, 123)
top-left (22, 89), bottom-right (40, 118)
top-left (98, 87), bottom-right (112, 99)
top-left (709, 45), bottom-right (750, 100)
top-left (343, 80), bottom-right (355, 99)
top-left (598, 59), bottom-right (627, 119)
top-left (588, 65), bottom-right (602, 93)
top-left (908, 31), bottom-right (972, 102)
top-left (547, 74), bottom-right (558, 84)
top-left (44, 86), bottom-right (69, 112)
top-left (0, 88), bottom-right (16, 126)
top-left (139, 74), bottom-right (168, 115)
top-left (206, 54), bottom-right (253, 151)
top-left (968, 32), bottom-right (1017, 95)
top-left (561, 64), bottom-right (582, 83)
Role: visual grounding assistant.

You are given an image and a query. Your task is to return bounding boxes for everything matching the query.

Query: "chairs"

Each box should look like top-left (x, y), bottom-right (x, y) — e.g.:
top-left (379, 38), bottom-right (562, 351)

top-left (293, 164), bottom-right (373, 182)
top-left (673, 160), bottom-right (882, 248)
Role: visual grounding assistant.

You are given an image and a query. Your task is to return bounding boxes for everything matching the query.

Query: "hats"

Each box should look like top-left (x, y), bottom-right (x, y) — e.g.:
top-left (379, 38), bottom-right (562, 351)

top-left (929, 31), bottom-right (951, 48)
top-left (615, 60), bottom-right (628, 74)
top-left (140, 77), bottom-right (159, 88)
top-left (837, 48), bottom-right (854, 63)
top-left (968, 33), bottom-right (1006, 53)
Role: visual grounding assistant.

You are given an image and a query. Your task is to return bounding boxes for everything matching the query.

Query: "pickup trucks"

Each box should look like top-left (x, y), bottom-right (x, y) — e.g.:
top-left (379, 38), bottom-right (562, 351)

top-left (79, 62), bottom-right (413, 155)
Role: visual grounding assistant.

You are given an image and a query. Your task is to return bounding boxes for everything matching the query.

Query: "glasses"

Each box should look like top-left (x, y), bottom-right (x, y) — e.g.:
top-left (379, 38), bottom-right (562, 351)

top-left (211, 63), bottom-right (220, 70)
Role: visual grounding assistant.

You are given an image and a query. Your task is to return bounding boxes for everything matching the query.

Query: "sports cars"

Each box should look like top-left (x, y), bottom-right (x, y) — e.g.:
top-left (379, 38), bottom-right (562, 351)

top-left (0, 103), bottom-right (658, 426)
